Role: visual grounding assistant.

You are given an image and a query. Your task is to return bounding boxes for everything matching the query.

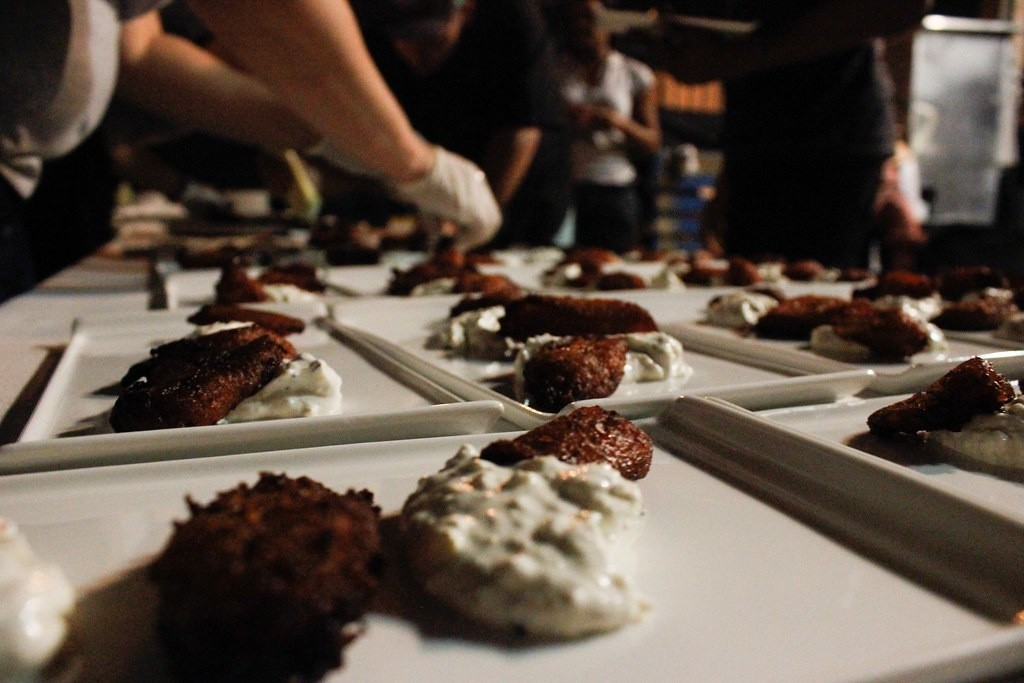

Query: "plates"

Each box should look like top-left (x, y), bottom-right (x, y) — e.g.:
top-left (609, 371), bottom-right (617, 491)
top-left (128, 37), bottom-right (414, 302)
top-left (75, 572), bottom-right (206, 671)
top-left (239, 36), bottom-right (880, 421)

top-left (0, 245), bottom-right (1024, 683)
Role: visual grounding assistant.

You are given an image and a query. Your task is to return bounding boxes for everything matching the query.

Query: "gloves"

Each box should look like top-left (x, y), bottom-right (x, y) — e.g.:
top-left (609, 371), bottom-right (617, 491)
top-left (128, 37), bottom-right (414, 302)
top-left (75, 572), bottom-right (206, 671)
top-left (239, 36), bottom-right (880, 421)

top-left (396, 146), bottom-right (503, 253)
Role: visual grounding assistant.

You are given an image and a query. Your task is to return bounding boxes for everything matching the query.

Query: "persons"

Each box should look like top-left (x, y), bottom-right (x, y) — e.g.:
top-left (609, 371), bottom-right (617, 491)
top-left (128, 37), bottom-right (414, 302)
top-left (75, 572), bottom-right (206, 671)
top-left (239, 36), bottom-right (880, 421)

top-left (0, 0), bottom-right (1023, 317)
